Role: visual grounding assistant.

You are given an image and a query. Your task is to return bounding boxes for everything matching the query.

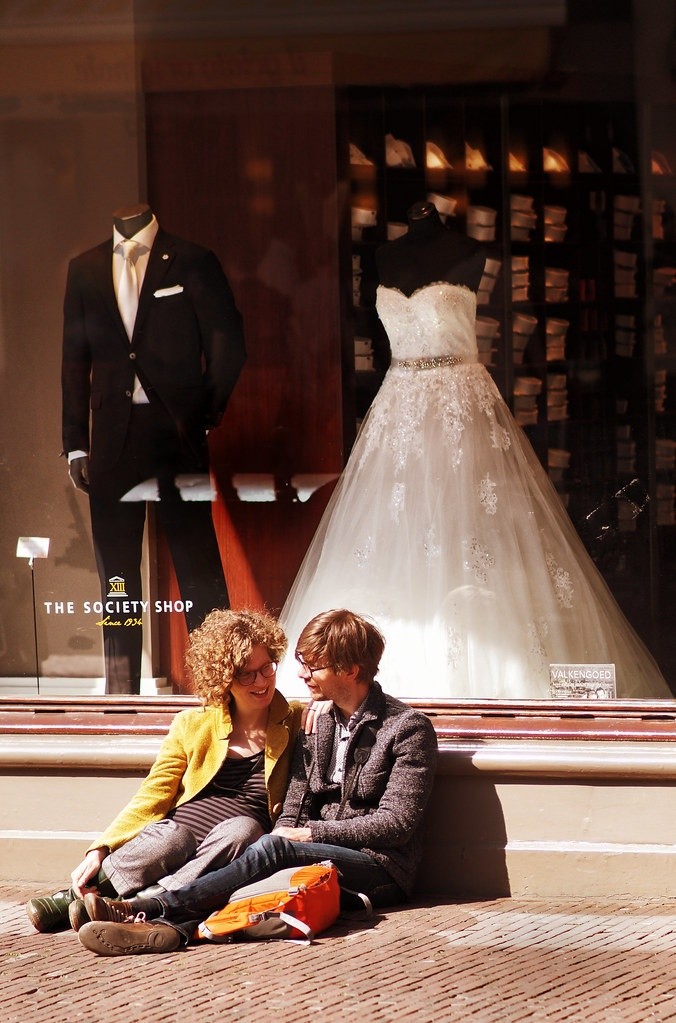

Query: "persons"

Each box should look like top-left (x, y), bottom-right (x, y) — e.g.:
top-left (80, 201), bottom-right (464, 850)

top-left (59, 205), bottom-right (246, 708)
top-left (78, 608), bottom-right (437, 956)
top-left (274, 199), bottom-right (673, 701)
top-left (26, 606), bottom-right (334, 933)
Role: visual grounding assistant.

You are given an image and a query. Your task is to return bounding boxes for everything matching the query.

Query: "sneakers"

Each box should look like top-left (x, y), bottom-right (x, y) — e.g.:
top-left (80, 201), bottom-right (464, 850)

top-left (76, 911), bottom-right (181, 957)
top-left (83, 892), bottom-right (138, 922)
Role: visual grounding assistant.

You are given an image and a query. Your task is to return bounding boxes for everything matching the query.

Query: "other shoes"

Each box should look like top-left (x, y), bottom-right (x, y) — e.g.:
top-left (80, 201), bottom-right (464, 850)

top-left (67, 894), bottom-right (127, 932)
top-left (26, 867), bottom-right (116, 933)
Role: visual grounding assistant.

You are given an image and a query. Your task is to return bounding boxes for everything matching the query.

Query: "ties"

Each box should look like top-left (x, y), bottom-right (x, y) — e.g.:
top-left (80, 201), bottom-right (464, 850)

top-left (119, 241), bottom-right (141, 343)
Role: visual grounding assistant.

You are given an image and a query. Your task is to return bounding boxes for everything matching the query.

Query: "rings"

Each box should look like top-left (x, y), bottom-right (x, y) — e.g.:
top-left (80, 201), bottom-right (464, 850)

top-left (306, 707), bottom-right (316, 711)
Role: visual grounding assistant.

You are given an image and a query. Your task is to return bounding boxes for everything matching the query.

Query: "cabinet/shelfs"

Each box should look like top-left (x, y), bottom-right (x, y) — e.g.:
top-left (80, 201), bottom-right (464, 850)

top-left (148, 80), bottom-right (675, 693)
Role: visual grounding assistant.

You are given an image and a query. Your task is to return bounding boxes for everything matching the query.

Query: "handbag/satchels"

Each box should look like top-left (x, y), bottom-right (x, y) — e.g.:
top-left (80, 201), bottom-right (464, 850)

top-left (191, 856), bottom-right (373, 946)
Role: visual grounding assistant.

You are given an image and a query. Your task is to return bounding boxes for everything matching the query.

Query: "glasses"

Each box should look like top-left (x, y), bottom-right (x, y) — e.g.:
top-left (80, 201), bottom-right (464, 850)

top-left (233, 658), bottom-right (279, 686)
top-left (294, 650), bottom-right (334, 678)
